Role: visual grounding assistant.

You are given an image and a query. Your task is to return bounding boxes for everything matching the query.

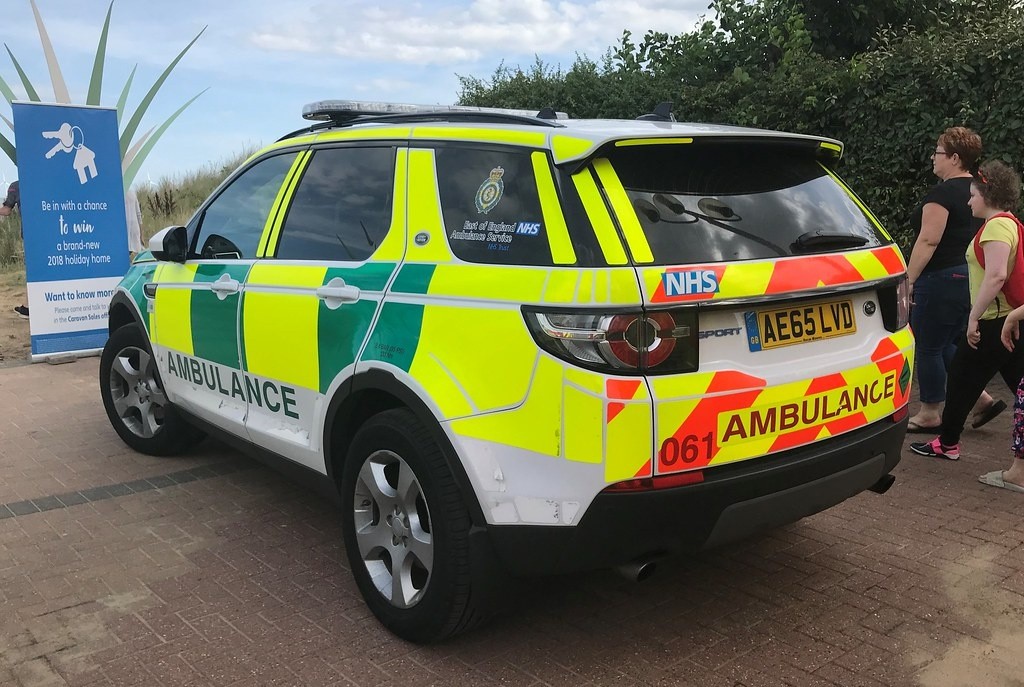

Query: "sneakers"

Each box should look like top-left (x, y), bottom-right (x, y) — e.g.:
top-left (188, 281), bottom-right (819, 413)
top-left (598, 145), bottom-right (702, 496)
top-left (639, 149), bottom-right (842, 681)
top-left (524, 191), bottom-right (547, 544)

top-left (909, 434), bottom-right (961, 460)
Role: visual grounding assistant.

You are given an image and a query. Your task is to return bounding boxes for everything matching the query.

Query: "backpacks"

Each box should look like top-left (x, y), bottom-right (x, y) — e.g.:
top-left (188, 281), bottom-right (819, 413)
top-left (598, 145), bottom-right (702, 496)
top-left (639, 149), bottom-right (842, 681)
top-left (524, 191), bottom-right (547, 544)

top-left (974, 212), bottom-right (1024, 309)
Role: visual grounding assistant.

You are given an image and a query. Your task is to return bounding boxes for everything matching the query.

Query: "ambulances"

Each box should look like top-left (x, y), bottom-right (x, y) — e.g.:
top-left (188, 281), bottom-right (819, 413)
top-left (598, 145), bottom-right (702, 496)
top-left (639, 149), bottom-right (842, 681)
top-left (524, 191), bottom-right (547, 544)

top-left (99, 99), bottom-right (915, 645)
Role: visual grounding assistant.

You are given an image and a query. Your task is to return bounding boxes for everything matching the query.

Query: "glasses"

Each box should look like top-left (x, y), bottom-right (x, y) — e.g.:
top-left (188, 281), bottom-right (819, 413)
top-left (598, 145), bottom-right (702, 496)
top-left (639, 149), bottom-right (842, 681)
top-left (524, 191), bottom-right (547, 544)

top-left (977, 167), bottom-right (998, 190)
top-left (933, 151), bottom-right (961, 158)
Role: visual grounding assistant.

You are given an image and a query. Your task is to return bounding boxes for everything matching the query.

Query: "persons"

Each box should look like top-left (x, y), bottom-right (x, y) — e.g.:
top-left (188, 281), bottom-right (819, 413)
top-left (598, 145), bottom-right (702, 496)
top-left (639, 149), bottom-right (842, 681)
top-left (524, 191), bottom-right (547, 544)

top-left (978, 305), bottom-right (1024, 492)
top-left (907, 127), bottom-right (1007, 433)
top-left (124, 191), bottom-right (145, 254)
top-left (42, 123), bottom-right (75, 159)
top-left (909, 160), bottom-right (1024, 460)
top-left (0, 179), bottom-right (29, 319)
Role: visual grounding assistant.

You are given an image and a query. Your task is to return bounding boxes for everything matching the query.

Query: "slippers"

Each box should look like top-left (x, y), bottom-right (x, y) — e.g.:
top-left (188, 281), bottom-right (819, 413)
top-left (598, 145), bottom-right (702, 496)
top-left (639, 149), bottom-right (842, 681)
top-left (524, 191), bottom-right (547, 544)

top-left (971, 399), bottom-right (1008, 429)
top-left (978, 470), bottom-right (1024, 493)
top-left (906, 415), bottom-right (943, 434)
